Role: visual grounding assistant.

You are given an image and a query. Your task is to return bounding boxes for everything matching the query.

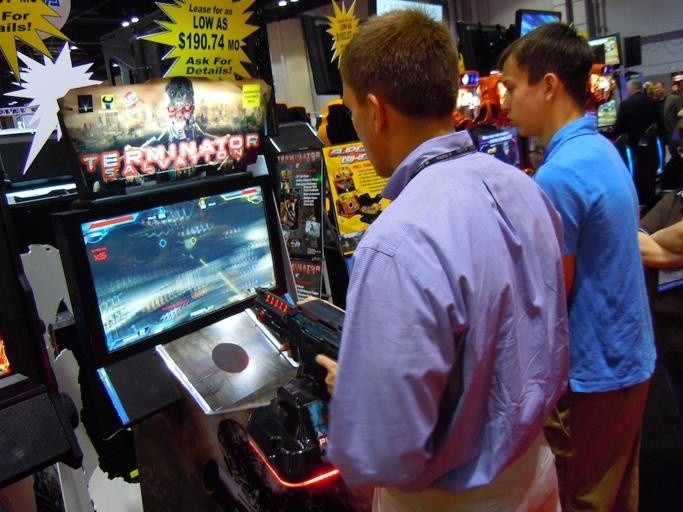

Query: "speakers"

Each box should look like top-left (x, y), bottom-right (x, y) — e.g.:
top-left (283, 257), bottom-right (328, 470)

top-left (624, 35), bottom-right (642, 67)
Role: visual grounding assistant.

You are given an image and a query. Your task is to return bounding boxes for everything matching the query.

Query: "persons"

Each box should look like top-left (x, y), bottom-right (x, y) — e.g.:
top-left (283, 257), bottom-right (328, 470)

top-left (495, 24), bottom-right (658, 512)
top-left (598, 74), bottom-right (682, 387)
top-left (312, 6), bottom-right (571, 512)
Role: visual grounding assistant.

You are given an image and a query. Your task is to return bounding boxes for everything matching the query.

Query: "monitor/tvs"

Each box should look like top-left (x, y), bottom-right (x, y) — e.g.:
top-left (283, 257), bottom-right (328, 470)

top-left (457, 20), bottom-right (506, 78)
top-left (515, 8), bottom-right (561, 40)
top-left (0, 326), bottom-right (17, 381)
top-left (366, 0), bottom-right (451, 33)
top-left (300, 12), bottom-right (368, 96)
top-left (597, 100), bottom-right (617, 128)
top-left (476, 125), bottom-right (521, 171)
top-left (587, 33), bottom-right (623, 66)
top-left (81, 185), bottom-right (279, 353)
